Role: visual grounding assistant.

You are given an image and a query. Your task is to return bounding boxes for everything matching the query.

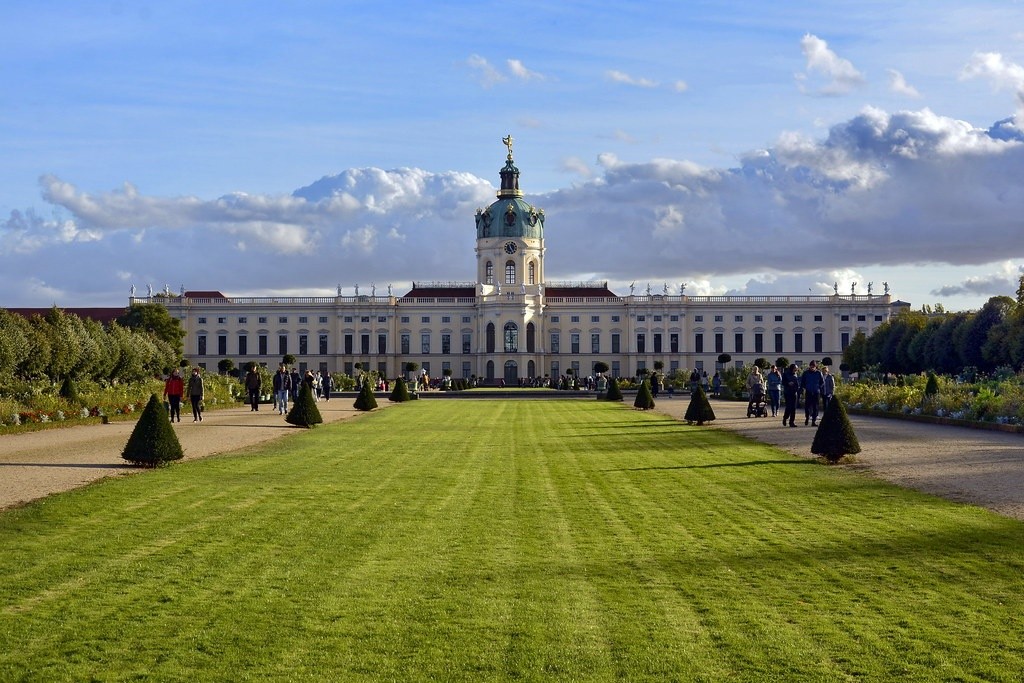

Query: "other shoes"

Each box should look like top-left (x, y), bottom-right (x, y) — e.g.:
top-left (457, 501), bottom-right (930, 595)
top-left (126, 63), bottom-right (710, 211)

top-left (199, 417), bottom-right (203, 422)
top-left (170, 420), bottom-right (174, 423)
top-left (655, 395), bottom-right (657, 398)
top-left (177, 419), bottom-right (181, 422)
top-left (285, 410), bottom-right (288, 415)
top-left (776, 412), bottom-right (779, 416)
top-left (255, 409), bottom-right (259, 411)
top-left (325, 400), bottom-right (329, 402)
top-left (251, 408), bottom-right (254, 411)
top-left (653, 395), bottom-right (655, 398)
top-left (783, 418), bottom-right (787, 426)
top-left (790, 422), bottom-right (797, 427)
top-left (273, 407), bottom-right (277, 411)
top-left (805, 418), bottom-right (810, 425)
top-left (316, 397), bottom-right (321, 402)
top-left (193, 419), bottom-right (198, 423)
top-left (810, 417), bottom-right (812, 420)
top-left (279, 411), bottom-right (283, 415)
top-left (816, 416), bottom-right (822, 420)
top-left (772, 414), bottom-right (776, 417)
top-left (812, 422), bottom-right (818, 427)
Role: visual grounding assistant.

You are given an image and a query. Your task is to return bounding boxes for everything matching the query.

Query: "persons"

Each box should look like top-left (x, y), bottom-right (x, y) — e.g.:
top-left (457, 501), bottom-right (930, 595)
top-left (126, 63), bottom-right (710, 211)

top-left (185, 368), bottom-right (204, 422)
top-left (712, 372), bottom-right (722, 396)
top-left (690, 368), bottom-right (701, 395)
top-left (419, 374), bottom-right (450, 391)
top-left (272, 370), bottom-right (281, 411)
top-left (701, 371), bottom-right (709, 395)
top-left (801, 360), bottom-right (824, 426)
top-left (163, 368), bottom-right (184, 424)
top-left (768, 365), bottom-right (782, 416)
top-left (305, 370), bottom-right (334, 401)
top-left (276, 366), bottom-right (292, 415)
top-left (375, 377), bottom-right (390, 391)
top-left (821, 366), bottom-right (834, 410)
top-left (745, 366), bottom-right (763, 395)
top-left (500, 378), bottom-right (505, 387)
top-left (244, 365), bottom-right (262, 411)
top-left (650, 372), bottom-right (659, 398)
top-left (517, 373), bottom-right (611, 392)
top-left (782, 364), bottom-right (799, 428)
top-left (667, 384), bottom-right (673, 398)
top-left (290, 368), bottom-right (302, 402)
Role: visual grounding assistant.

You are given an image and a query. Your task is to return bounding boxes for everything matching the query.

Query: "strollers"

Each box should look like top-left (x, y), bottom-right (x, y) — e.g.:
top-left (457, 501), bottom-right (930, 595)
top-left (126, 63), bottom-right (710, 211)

top-left (690, 381), bottom-right (699, 398)
top-left (747, 383), bottom-right (768, 418)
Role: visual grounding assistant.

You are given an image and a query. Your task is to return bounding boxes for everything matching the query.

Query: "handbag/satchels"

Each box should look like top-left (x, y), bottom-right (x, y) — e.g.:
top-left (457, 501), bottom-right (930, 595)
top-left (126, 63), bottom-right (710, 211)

top-left (197, 405), bottom-right (205, 412)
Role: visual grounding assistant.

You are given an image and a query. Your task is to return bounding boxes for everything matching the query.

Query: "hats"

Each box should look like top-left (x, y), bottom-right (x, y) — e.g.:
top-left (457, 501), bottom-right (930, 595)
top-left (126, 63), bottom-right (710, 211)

top-left (809, 360), bottom-right (817, 367)
top-left (771, 365), bottom-right (776, 369)
